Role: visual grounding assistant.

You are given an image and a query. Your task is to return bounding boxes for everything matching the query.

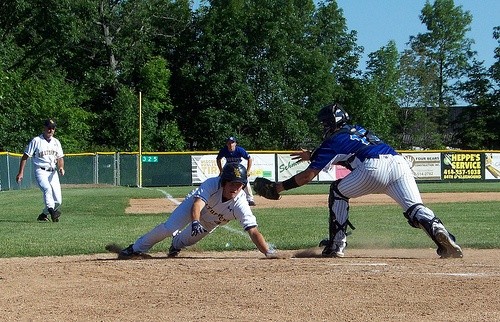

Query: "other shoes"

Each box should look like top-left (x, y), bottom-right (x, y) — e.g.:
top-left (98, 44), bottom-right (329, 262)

top-left (167, 241), bottom-right (181, 256)
top-left (121, 244), bottom-right (138, 256)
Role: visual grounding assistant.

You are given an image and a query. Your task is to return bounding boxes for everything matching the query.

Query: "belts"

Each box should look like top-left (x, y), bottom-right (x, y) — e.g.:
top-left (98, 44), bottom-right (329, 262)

top-left (41, 167), bottom-right (57, 172)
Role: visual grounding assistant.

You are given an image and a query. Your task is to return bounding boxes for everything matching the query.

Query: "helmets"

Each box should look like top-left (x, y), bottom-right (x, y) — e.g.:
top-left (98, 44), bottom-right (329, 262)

top-left (220, 162), bottom-right (248, 190)
top-left (317, 104), bottom-right (350, 140)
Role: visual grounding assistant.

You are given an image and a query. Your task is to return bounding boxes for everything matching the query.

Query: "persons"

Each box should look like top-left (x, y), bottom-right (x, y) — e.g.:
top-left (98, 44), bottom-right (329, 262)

top-left (253, 103), bottom-right (464, 259)
top-left (117, 162), bottom-right (276, 259)
top-left (216, 137), bottom-right (256, 206)
top-left (17, 119), bottom-right (65, 222)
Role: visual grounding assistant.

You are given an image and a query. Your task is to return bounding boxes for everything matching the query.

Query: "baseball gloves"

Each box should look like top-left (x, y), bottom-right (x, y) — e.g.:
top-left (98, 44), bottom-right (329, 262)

top-left (254, 177), bottom-right (282, 200)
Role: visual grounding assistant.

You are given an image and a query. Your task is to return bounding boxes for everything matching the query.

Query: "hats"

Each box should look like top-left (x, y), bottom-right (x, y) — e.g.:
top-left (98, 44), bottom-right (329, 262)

top-left (226, 137), bottom-right (237, 143)
top-left (44, 119), bottom-right (57, 128)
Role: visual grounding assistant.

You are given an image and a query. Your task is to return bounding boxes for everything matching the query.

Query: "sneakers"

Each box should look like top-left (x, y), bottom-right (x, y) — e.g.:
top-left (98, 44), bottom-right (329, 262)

top-left (38, 213), bottom-right (50, 221)
top-left (435, 228), bottom-right (464, 259)
top-left (320, 239), bottom-right (344, 257)
top-left (51, 209), bottom-right (61, 222)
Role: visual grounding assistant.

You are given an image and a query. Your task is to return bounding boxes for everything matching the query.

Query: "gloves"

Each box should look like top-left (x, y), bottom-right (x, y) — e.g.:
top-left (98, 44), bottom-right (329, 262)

top-left (192, 221), bottom-right (207, 236)
top-left (265, 249), bottom-right (276, 256)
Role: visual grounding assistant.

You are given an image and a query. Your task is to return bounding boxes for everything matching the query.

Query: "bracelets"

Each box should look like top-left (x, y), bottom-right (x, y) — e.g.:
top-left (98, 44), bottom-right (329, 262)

top-left (282, 176), bottom-right (300, 191)
